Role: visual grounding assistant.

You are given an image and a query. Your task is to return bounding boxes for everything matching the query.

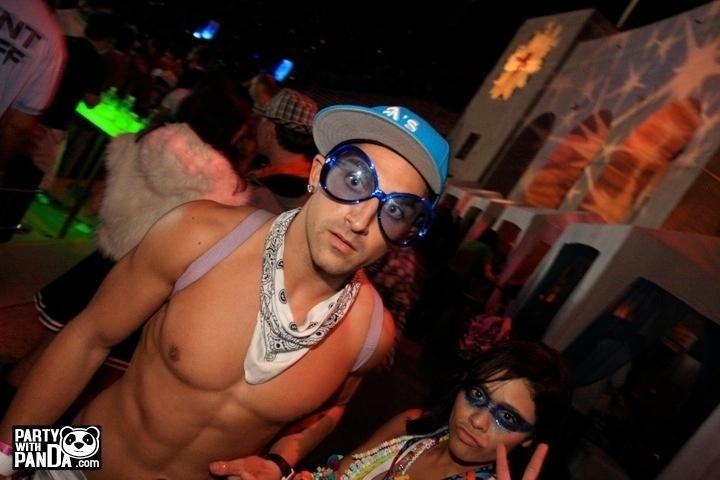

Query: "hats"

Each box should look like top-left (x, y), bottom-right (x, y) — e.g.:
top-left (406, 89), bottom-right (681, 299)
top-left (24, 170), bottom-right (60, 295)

top-left (312, 101), bottom-right (452, 200)
top-left (250, 89), bottom-right (319, 130)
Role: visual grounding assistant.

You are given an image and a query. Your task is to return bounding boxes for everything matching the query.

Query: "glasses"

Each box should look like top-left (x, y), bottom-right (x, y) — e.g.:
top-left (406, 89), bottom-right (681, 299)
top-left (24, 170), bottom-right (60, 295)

top-left (318, 144), bottom-right (433, 247)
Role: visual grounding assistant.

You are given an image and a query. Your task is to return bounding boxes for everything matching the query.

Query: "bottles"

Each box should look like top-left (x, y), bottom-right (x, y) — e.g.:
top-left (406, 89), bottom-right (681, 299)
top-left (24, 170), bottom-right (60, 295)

top-left (101, 86), bottom-right (136, 111)
